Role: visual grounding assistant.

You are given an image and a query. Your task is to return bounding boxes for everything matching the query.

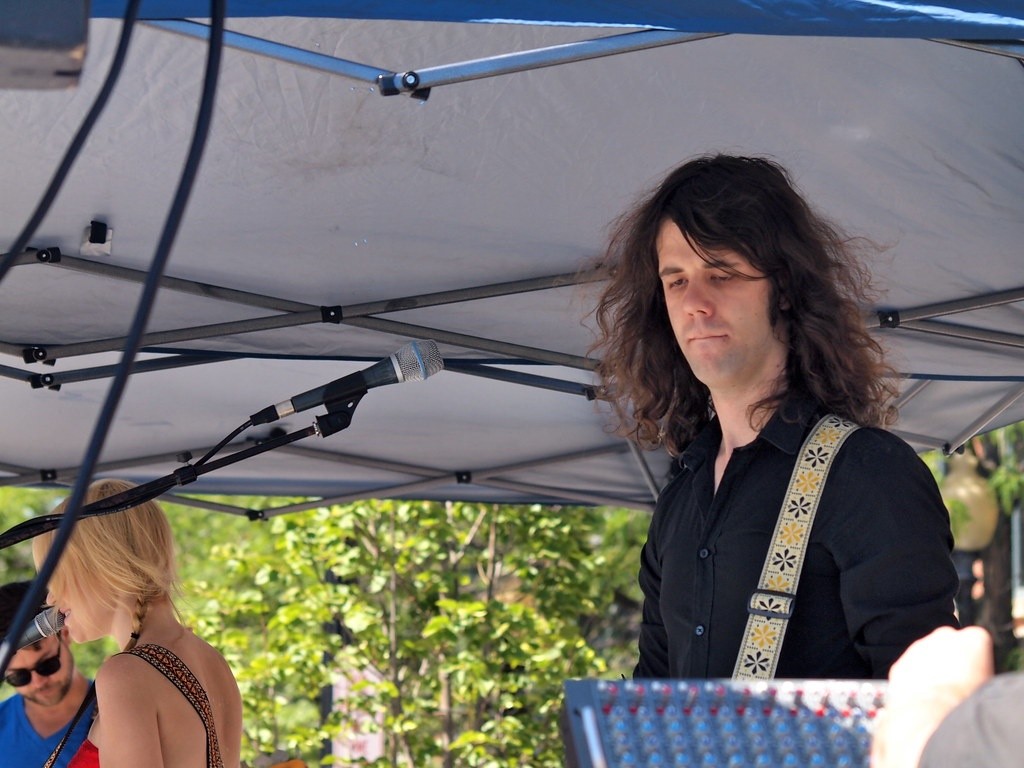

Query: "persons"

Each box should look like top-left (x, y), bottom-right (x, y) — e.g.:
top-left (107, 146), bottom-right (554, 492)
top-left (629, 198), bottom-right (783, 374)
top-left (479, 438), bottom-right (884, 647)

top-left (582, 155), bottom-right (959, 679)
top-left (32, 479), bottom-right (243, 768)
top-left (0, 581), bottom-right (96, 768)
top-left (868, 626), bottom-right (1024, 768)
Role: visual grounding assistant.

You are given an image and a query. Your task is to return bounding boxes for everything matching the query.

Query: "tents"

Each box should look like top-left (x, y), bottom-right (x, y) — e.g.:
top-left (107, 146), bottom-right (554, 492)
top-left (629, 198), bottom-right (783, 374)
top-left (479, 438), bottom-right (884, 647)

top-left (0, 0), bottom-right (1024, 676)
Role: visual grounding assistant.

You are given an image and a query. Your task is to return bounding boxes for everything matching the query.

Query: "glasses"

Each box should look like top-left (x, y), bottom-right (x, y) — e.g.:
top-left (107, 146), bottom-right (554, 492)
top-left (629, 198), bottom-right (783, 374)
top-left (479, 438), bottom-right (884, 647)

top-left (1, 638), bottom-right (63, 689)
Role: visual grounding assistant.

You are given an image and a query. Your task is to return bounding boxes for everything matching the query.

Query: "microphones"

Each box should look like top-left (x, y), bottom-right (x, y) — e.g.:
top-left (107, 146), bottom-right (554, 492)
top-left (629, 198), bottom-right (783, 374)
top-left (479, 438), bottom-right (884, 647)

top-left (17, 608), bottom-right (65, 649)
top-left (251, 339), bottom-right (445, 426)
